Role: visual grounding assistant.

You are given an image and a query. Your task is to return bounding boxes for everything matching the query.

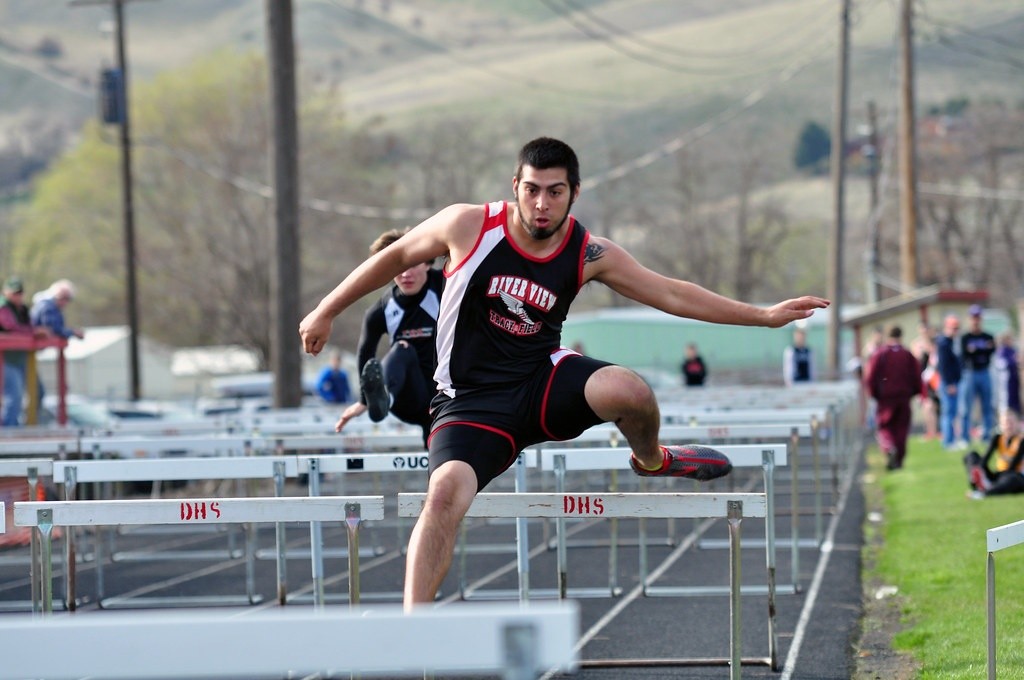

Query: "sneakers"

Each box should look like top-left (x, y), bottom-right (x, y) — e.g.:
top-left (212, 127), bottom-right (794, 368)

top-left (628, 444), bottom-right (732, 482)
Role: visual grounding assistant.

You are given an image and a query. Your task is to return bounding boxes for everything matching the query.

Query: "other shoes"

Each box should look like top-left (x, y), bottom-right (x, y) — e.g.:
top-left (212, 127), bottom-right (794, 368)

top-left (967, 490), bottom-right (985, 501)
top-left (359, 358), bottom-right (391, 423)
top-left (972, 467), bottom-right (992, 494)
top-left (887, 452), bottom-right (901, 469)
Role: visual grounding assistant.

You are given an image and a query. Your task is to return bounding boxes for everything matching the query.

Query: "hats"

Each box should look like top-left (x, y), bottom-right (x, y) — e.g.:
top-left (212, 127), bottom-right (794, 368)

top-left (967, 305), bottom-right (982, 317)
top-left (2, 277), bottom-right (25, 294)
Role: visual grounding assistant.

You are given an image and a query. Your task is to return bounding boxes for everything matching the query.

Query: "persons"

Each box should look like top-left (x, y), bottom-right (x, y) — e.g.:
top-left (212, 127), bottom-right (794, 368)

top-left (336, 227), bottom-right (446, 452)
top-left (0, 278), bottom-right (85, 426)
top-left (315, 353), bottom-right (351, 403)
top-left (297, 136), bottom-right (830, 611)
top-left (782, 330), bottom-right (815, 385)
top-left (860, 305), bottom-right (1024, 501)
top-left (681, 344), bottom-right (709, 386)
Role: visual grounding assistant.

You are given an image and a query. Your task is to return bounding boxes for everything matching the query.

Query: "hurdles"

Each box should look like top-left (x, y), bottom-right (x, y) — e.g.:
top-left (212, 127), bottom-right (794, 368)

top-left (987, 520), bottom-right (1024, 680)
top-left (0, 382), bottom-right (862, 680)
